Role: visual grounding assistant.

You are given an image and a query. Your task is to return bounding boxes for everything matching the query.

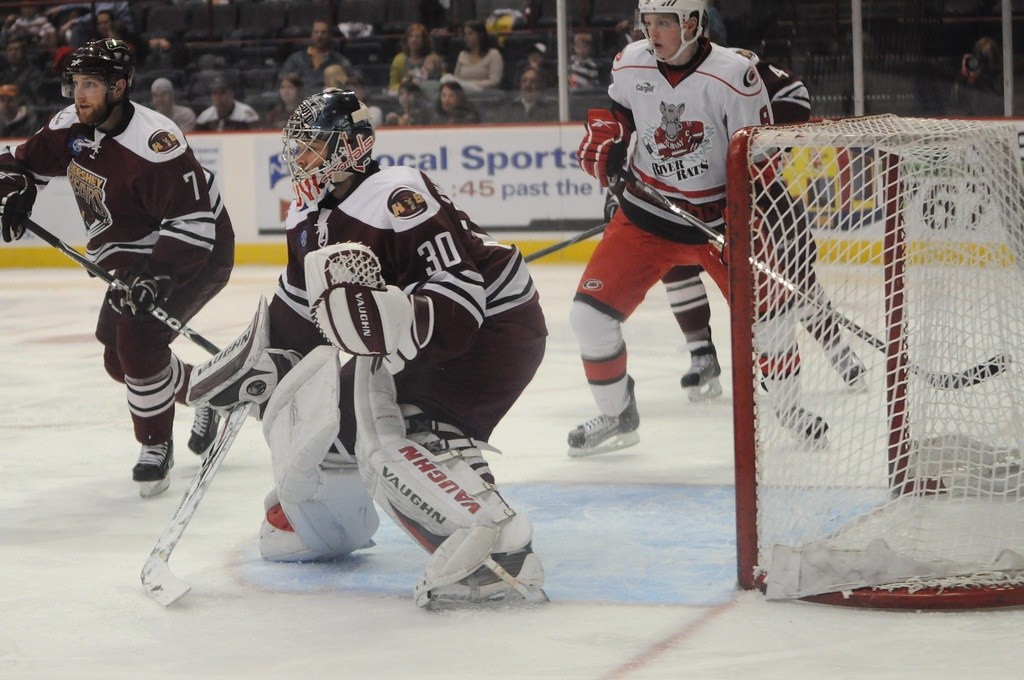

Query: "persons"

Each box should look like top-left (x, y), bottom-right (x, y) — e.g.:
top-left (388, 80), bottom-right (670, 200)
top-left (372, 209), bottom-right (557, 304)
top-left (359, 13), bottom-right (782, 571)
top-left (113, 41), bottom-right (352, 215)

top-left (386, 6), bottom-right (600, 126)
top-left (660, 8), bottom-right (865, 399)
top-left (191, 86), bottom-right (547, 606)
top-left (567, 0), bottom-right (829, 458)
top-left (266, 19), bottom-right (384, 128)
top-left (0, 39), bottom-right (235, 498)
top-left (196, 78), bottom-right (260, 131)
top-left (0, 0), bottom-right (148, 138)
top-left (151, 77), bottom-right (195, 131)
top-left (954, 37), bottom-right (1004, 115)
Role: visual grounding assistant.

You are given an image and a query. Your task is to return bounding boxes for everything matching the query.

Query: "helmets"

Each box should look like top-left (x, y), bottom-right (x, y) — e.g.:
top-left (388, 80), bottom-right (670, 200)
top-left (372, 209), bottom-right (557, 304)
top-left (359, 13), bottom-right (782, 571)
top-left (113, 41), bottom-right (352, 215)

top-left (64, 39), bottom-right (135, 88)
top-left (281, 88), bottom-right (375, 204)
top-left (638, 1), bottom-right (705, 60)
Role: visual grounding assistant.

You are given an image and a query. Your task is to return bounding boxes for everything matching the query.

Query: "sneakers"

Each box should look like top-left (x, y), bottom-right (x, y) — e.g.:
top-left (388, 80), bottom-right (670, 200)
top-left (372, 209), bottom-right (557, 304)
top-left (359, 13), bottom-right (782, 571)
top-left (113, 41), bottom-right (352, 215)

top-left (828, 347), bottom-right (867, 390)
top-left (132, 442), bottom-right (174, 497)
top-left (567, 375), bottom-right (640, 457)
top-left (777, 405), bottom-right (825, 448)
top-left (680, 339), bottom-right (722, 404)
top-left (188, 407), bottom-right (222, 461)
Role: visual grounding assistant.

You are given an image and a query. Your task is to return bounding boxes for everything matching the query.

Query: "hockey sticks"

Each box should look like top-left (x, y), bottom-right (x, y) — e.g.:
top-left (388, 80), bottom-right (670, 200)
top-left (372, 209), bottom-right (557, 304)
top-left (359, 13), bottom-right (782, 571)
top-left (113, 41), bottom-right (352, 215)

top-left (20, 216), bottom-right (220, 356)
top-left (524, 222), bottom-right (607, 264)
top-left (611, 158), bottom-right (1013, 392)
top-left (140, 401), bottom-right (255, 607)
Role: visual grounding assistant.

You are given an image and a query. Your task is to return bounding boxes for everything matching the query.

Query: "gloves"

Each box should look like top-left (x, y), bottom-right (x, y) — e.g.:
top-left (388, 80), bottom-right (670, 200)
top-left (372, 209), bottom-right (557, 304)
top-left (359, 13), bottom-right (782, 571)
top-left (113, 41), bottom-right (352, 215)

top-left (577, 107), bottom-right (630, 185)
top-left (106, 261), bottom-right (175, 321)
top-left (0, 161), bottom-right (36, 242)
top-left (719, 204), bottom-right (768, 273)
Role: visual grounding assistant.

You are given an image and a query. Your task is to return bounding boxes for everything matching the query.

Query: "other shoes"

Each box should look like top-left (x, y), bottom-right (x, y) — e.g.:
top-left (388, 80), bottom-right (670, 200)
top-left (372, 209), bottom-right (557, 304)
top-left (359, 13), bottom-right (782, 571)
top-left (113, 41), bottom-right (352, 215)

top-left (259, 499), bottom-right (377, 562)
top-left (416, 544), bottom-right (547, 613)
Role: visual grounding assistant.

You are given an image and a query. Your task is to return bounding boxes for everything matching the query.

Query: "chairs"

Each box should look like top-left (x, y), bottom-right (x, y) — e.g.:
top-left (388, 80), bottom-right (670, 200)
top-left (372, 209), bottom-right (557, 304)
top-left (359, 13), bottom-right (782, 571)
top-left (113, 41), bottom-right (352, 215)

top-left (33, 0), bottom-right (1024, 118)
